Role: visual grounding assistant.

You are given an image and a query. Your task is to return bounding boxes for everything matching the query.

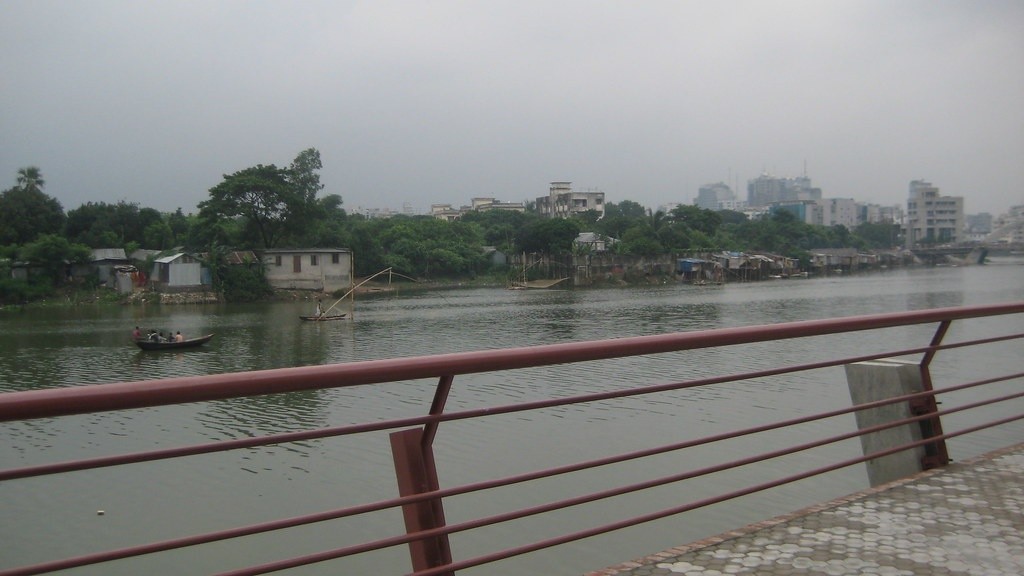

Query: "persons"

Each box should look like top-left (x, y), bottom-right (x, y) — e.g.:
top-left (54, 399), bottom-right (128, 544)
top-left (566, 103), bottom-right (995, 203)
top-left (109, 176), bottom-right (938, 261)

top-left (133, 327), bottom-right (142, 336)
top-left (317, 299), bottom-right (321, 314)
top-left (148, 330), bottom-right (182, 342)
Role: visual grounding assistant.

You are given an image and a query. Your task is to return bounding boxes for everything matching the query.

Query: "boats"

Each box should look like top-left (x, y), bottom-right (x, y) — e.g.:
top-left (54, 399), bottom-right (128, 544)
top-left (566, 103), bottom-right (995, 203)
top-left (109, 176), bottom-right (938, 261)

top-left (299, 314), bottom-right (347, 321)
top-left (131, 333), bottom-right (215, 351)
